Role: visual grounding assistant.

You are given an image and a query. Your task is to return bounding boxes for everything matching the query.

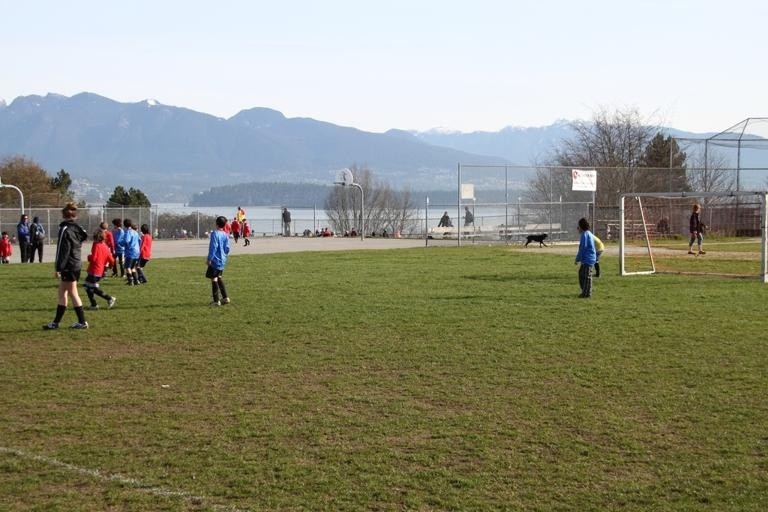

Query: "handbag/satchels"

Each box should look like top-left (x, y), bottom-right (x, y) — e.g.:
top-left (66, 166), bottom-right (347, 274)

top-left (35, 231), bottom-right (45, 241)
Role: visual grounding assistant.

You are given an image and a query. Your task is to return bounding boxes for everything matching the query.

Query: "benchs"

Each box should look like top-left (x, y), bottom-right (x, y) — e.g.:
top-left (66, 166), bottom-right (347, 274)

top-left (605, 220), bottom-right (680, 241)
top-left (425, 222), bottom-right (569, 247)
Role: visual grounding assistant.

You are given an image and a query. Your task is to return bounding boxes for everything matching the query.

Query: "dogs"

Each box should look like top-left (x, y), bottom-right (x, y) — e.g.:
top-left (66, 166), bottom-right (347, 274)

top-left (525, 234), bottom-right (548, 248)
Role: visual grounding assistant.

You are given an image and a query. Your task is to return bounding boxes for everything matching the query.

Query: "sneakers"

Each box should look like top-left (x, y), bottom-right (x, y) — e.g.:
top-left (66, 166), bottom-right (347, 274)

top-left (108, 296), bottom-right (116, 309)
top-left (209, 300), bottom-right (221, 306)
top-left (220, 297), bottom-right (230, 304)
top-left (84, 305), bottom-right (99, 310)
top-left (111, 274), bottom-right (147, 286)
top-left (69, 321), bottom-right (89, 329)
top-left (43, 322), bottom-right (59, 330)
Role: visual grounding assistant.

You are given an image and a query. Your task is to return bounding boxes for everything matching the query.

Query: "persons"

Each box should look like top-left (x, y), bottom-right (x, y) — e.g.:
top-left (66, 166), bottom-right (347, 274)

top-left (688, 204), bottom-right (706, 256)
top-left (438, 211), bottom-right (453, 235)
top-left (577, 227), bottom-right (605, 277)
top-left (464, 207), bottom-right (473, 238)
top-left (577, 218), bottom-right (593, 298)
top-left (0, 203), bottom-right (254, 328)
top-left (282, 208), bottom-right (291, 236)
top-left (303, 227), bottom-right (389, 237)
